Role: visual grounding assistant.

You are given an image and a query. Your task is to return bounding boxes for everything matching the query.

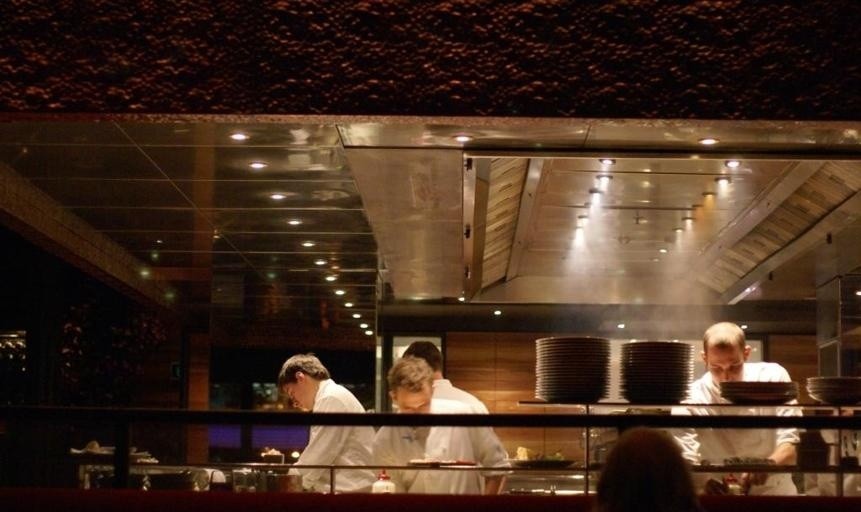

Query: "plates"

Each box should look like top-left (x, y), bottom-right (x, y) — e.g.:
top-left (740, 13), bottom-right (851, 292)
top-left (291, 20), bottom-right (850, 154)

top-left (509, 459), bottom-right (578, 467)
top-left (618, 340), bottom-right (694, 404)
top-left (532, 336), bottom-right (610, 402)
top-left (806, 376), bottom-right (861, 405)
top-left (718, 379), bottom-right (800, 404)
top-left (72, 447), bottom-right (149, 457)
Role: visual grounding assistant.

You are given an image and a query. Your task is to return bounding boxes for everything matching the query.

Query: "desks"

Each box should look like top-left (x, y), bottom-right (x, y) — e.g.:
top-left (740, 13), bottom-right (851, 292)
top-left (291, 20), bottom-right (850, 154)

top-left (80, 465), bottom-right (226, 490)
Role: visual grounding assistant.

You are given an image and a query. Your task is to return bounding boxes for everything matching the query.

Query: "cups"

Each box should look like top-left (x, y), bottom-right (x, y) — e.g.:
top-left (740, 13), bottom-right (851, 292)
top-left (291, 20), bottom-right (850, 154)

top-left (263, 452), bottom-right (285, 464)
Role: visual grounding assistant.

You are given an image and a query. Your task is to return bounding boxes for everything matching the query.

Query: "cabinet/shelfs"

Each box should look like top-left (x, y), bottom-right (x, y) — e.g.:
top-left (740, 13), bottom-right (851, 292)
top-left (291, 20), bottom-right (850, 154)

top-left (815, 273), bottom-right (861, 376)
top-left (406, 401), bottom-right (861, 474)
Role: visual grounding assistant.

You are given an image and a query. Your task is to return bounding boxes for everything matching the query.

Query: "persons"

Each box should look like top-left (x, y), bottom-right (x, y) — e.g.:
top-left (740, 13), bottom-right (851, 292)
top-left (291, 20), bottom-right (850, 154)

top-left (667, 323), bottom-right (802, 500)
top-left (375, 353), bottom-right (516, 495)
top-left (273, 352), bottom-right (375, 494)
top-left (594, 426), bottom-right (700, 507)
top-left (401, 339), bottom-right (490, 413)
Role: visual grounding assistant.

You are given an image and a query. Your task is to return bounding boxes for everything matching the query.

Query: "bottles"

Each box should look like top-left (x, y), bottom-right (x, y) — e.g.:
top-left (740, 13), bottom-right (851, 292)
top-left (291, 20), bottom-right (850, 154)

top-left (726, 472), bottom-right (743, 495)
top-left (372, 469), bottom-right (396, 493)
